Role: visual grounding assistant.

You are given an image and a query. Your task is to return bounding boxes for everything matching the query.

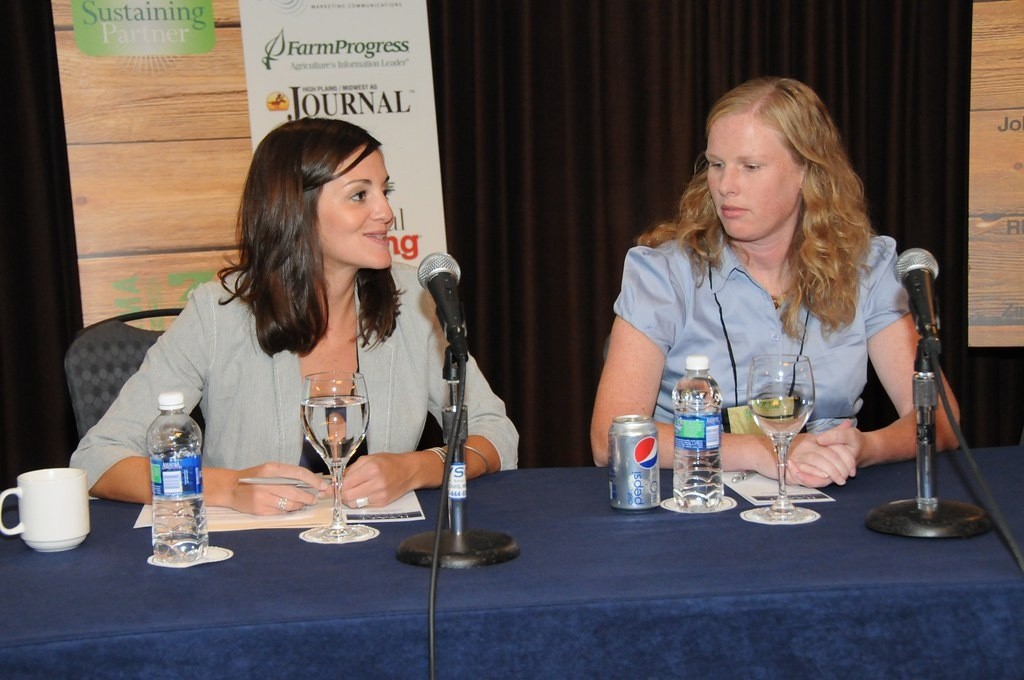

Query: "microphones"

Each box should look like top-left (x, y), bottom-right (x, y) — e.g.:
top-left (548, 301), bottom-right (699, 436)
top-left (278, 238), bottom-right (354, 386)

top-left (896, 248), bottom-right (941, 358)
top-left (417, 251), bottom-right (468, 362)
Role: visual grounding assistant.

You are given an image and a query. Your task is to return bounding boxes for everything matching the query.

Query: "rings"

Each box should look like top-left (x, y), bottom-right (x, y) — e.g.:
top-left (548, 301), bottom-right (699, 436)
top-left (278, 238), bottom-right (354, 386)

top-left (275, 498), bottom-right (286, 512)
top-left (353, 496), bottom-right (371, 510)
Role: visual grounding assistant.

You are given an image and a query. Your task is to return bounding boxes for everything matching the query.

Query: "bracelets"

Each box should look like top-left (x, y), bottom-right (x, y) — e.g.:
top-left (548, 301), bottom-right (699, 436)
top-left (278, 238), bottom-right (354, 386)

top-left (459, 443), bottom-right (490, 476)
top-left (424, 446), bottom-right (448, 466)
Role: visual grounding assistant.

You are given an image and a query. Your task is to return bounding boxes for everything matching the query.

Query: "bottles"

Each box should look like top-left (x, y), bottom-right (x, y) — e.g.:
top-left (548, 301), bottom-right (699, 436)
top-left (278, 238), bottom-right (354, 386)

top-left (144, 389), bottom-right (211, 566)
top-left (671, 354), bottom-right (725, 511)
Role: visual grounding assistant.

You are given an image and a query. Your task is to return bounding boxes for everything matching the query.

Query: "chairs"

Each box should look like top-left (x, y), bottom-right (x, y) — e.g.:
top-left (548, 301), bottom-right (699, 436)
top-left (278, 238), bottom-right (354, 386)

top-left (63, 309), bottom-right (187, 442)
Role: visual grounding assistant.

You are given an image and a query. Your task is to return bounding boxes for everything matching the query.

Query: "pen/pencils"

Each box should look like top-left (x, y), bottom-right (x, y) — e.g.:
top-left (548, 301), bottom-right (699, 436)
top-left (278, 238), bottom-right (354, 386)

top-left (730, 469), bottom-right (758, 484)
top-left (238, 476), bottom-right (314, 489)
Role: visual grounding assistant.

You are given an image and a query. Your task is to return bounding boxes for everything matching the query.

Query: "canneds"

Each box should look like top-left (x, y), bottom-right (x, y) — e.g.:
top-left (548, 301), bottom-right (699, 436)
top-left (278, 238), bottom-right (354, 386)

top-left (607, 415), bottom-right (661, 514)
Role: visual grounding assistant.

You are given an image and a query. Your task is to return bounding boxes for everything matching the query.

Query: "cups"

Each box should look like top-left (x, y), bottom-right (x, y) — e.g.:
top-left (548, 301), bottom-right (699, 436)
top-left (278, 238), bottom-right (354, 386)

top-left (0, 465), bottom-right (92, 554)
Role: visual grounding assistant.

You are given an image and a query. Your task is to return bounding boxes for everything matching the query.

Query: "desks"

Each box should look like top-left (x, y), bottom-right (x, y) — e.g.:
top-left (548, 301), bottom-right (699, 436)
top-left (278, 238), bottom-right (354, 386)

top-left (1, 444), bottom-right (1023, 679)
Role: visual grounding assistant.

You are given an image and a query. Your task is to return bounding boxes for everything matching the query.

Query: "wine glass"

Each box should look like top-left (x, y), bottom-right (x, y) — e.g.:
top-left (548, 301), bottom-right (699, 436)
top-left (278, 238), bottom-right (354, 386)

top-left (748, 352), bottom-right (816, 523)
top-left (300, 371), bottom-right (373, 544)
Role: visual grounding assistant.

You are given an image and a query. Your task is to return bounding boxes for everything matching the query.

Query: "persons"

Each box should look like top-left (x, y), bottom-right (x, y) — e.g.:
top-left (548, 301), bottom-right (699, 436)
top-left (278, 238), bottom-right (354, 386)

top-left (590, 75), bottom-right (962, 489)
top-left (66, 116), bottom-right (522, 516)
